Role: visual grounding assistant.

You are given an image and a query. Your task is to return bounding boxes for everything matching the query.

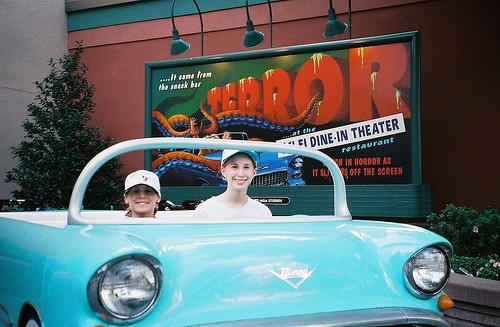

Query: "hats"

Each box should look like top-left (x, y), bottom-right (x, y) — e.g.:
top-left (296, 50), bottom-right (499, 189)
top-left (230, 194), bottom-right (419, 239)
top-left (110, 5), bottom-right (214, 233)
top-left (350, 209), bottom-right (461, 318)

top-left (221, 149), bottom-right (259, 168)
top-left (124, 170), bottom-right (161, 197)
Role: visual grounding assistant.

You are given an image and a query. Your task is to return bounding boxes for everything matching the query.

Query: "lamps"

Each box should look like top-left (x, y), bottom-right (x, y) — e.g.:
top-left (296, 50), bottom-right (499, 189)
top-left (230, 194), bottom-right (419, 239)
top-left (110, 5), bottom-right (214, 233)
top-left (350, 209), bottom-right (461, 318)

top-left (243, 0), bottom-right (273, 49)
top-left (170, 0), bottom-right (205, 57)
top-left (322, 0), bottom-right (352, 40)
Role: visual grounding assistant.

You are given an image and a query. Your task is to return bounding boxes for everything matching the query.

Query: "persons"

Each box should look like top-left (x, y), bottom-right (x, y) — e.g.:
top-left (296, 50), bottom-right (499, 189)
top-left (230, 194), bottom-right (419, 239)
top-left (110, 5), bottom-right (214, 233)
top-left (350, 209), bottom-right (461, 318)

top-left (123, 169), bottom-right (162, 218)
top-left (222, 130), bottom-right (232, 140)
top-left (192, 149), bottom-right (273, 219)
top-left (189, 117), bottom-right (220, 157)
